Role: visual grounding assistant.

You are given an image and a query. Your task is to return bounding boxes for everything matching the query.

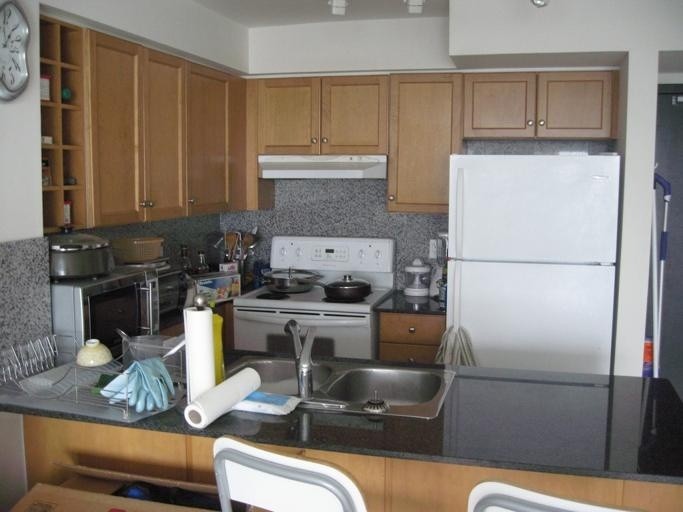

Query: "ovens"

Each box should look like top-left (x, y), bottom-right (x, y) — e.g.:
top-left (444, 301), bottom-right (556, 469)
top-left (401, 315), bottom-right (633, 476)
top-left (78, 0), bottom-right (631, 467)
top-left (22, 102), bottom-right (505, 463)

top-left (233, 308), bottom-right (380, 359)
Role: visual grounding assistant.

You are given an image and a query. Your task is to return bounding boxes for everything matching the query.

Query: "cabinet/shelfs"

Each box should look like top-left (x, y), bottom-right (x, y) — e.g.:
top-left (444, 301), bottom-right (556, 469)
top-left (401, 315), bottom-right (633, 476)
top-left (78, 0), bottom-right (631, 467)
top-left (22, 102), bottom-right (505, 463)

top-left (184, 61), bottom-right (246, 217)
top-left (460, 71), bottom-right (619, 140)
top-left (378, 311), bottom-right (447, 363)
top-left (385, 71), bottom-right (460, 214)
top-left (40, 13), bottom-right (87, 236)
top-left (87, 26), bottom-right (186, 228)
top-left (257, 75), bottom-right (391, 155)
top-left (157, 303), bottom-right (232, 352)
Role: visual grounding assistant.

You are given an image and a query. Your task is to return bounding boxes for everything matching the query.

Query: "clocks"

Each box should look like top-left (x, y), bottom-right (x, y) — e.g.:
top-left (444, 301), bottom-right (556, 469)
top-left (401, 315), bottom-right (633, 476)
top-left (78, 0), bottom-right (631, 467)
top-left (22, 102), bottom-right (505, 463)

top-left (0, 1), bottom-right (30, 102)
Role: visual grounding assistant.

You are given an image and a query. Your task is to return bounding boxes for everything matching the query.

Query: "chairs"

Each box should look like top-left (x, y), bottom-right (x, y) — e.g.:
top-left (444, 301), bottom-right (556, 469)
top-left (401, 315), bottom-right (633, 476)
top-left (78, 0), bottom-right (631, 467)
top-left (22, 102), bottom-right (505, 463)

top-left (210, 434), bottom-right (367, 512)
top-left (465, 479), bottom-right (626, 512)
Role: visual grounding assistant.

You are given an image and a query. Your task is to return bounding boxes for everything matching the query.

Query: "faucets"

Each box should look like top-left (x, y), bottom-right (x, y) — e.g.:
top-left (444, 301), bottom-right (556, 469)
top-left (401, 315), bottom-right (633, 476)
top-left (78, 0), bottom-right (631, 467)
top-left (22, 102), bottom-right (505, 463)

top-left (284, 319), bottom-right (319, 398)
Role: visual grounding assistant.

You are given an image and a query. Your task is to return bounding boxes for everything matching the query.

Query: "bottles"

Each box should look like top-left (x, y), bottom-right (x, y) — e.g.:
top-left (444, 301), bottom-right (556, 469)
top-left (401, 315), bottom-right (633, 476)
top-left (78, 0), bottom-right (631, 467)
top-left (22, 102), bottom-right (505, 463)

top-left (178, 244), bottom-right (194, 275)
top-left (196, 249), bottom-right (210, 277)
top-left (210, 300), bottom-right (225, 385)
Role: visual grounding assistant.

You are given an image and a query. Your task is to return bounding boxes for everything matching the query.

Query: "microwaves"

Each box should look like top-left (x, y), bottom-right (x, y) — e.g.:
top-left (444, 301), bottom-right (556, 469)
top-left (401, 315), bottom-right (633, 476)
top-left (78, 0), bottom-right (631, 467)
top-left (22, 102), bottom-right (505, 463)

top-left (157, 271), bottom-right (188, 319)
top-left (52, 267), bottom-right (159, 366)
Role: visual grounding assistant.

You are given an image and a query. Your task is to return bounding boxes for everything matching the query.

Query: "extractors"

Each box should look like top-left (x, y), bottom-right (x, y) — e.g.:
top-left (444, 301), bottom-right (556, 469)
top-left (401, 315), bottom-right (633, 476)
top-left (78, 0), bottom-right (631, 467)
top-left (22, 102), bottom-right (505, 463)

top-left (257, 153), bottom-right (387, 180)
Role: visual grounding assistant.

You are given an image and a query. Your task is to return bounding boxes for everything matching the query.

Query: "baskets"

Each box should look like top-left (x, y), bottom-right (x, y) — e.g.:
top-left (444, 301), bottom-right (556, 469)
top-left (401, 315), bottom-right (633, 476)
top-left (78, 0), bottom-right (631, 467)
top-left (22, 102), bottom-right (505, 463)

top-left (110, 237), bottom-right (164, 263)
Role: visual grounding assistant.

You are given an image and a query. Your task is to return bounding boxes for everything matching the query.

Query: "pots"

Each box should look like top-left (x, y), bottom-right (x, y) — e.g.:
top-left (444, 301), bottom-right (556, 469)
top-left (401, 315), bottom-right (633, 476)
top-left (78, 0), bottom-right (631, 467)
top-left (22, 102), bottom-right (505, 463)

top-left (49, 223), bottom-right (115, 283)
top-left (260, 269), bottom-right (323, 294)
top-left (298, 274), bottom-right (371, 301)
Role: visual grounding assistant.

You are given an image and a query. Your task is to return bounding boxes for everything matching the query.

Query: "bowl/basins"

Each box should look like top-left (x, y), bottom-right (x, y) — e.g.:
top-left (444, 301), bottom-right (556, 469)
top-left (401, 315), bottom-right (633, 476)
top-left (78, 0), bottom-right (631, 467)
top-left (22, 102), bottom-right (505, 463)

top-left (77, 338), bottom-right (112, 366)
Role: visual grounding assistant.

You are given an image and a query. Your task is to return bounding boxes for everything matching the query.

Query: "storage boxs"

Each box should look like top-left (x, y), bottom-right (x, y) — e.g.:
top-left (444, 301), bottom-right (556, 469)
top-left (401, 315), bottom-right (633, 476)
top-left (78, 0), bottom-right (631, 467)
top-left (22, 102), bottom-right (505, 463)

top-left (122, 333), bottom-right (185, 384)
top-left (186, 270), bottom-right (241, 308)
top-left (9, 465), bottom-right (253, 512)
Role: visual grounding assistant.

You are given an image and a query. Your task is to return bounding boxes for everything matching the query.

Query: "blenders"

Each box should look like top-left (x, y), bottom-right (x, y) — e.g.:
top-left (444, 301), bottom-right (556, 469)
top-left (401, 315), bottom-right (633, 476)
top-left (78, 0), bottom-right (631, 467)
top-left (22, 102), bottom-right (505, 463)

top-left (403, 238), bottom-right (447, 297)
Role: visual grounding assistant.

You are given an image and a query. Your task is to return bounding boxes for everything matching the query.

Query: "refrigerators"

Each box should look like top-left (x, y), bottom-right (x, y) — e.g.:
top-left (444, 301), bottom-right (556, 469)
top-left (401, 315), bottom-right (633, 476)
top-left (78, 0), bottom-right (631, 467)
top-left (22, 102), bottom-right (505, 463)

top-left (446, 155), bottom-right (622, 375)
top-left (442, 367), bottom-right (611, 471)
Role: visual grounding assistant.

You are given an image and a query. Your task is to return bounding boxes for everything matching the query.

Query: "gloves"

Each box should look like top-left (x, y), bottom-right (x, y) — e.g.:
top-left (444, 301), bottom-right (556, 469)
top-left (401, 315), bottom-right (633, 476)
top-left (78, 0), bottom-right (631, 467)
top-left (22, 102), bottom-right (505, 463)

top-left (101, 358), bottom-right (175, 412)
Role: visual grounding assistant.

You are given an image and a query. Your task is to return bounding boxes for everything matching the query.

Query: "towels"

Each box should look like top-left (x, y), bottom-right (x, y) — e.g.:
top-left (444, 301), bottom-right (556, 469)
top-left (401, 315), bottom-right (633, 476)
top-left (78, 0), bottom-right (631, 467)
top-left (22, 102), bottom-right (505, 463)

top-left (434, 326), bottom-right (474, 368)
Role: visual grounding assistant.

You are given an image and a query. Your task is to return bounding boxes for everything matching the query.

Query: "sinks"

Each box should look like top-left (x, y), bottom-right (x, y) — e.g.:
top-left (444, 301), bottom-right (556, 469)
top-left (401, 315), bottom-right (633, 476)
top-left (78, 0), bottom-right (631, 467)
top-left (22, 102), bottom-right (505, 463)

top-left (326, 362), bottom-right (456, 421)
top-left (224, 355), bottom-right (333, 402)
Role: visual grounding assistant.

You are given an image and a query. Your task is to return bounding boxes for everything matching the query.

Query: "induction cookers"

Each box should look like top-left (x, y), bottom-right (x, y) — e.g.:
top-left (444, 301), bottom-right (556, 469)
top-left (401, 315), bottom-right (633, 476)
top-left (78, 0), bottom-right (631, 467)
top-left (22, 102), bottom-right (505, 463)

top-left (232, 235), bottom-right (396, 312)
